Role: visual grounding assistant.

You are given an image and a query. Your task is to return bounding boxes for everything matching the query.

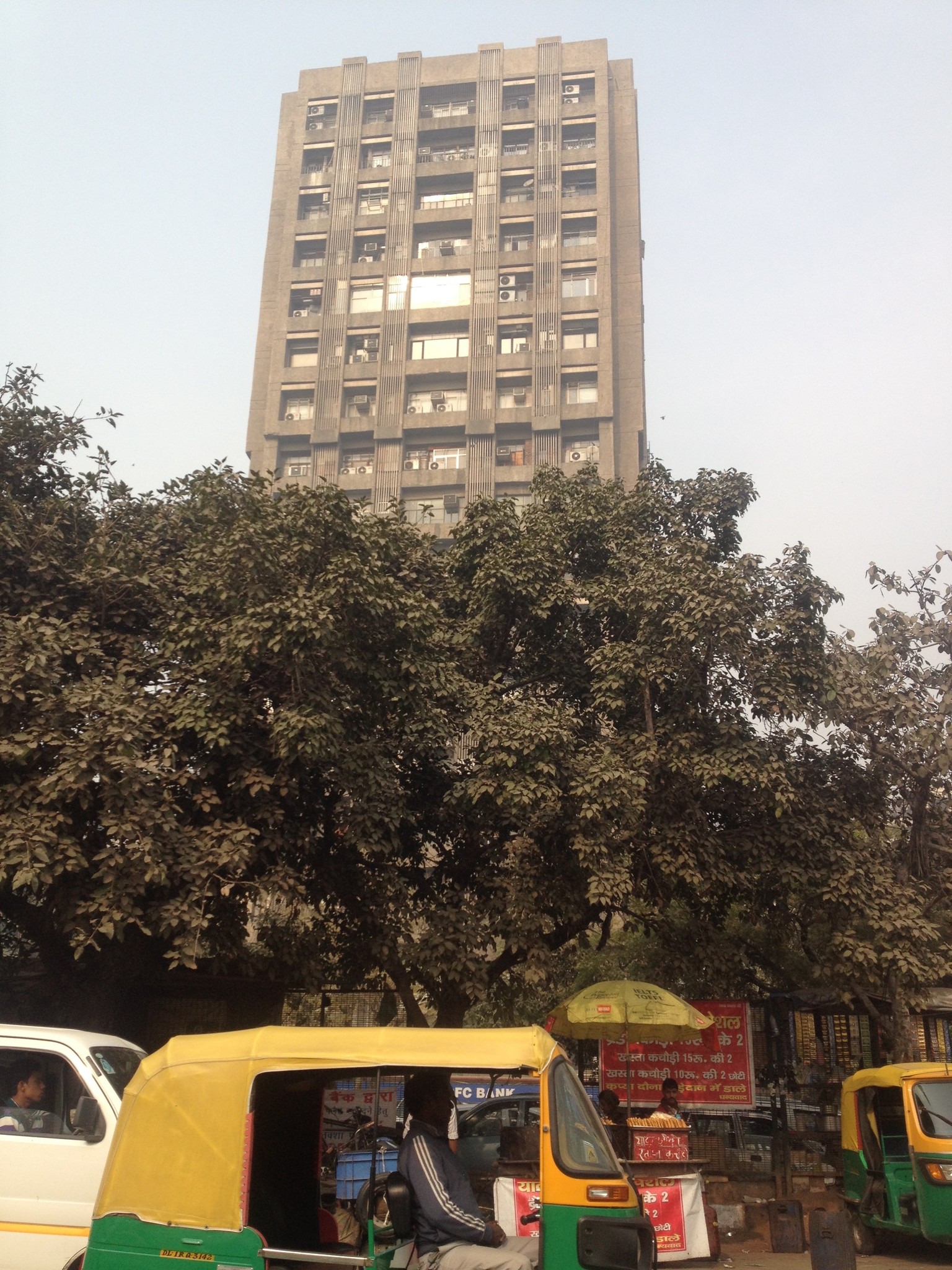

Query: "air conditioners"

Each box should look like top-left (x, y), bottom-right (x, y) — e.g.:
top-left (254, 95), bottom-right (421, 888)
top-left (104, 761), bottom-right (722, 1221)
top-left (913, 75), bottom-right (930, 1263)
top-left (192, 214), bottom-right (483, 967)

top-left (428, 461), bottom-right (445, 470)
top-left (358, 240), bottom-right (455, 262)
top-left (292, 288), bottom-right (321, 316)
top-left (495, 446), bottom-right (512, 461)
top-left (323, 194), bottom-right (329, 210)
top-left (499, 275), bottom-right (516, 302)
top-left (417, 99), bottom-right (477, 160)
top-left (284, 412), bottom-right (300, 421)
top-left (306, 107), bottom-right (394, 132)
top-left (288, 466), bottom-right (303, 476)
top-left (350, 338), bottom-right (379, 363)
top-left (513, 387), bottom-right (526, 402)
top-left (408, 391), bottom-right (453, 414)
top-left (403, 460), bottom-right (421, 470)
top-left (354, 395), bottom-right (370, 410)
top-left (570, 451), bottom-right (587, 463)
top-left (539, 84), bottom-right (580, 151)
top-left (443, 494), bottom-right (460, 508)
top-left (477, 340), bottom-right (555, 354)
top-left (340, 466), bottom-right (374, 476)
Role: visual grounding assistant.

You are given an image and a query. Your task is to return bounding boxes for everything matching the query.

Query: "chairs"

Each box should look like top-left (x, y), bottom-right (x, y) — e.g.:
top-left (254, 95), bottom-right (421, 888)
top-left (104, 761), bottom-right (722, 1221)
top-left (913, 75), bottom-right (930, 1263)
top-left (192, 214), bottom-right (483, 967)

top-left (388, 1171), bottom-right (416, 1238)
top-left (496, 1126), bottom-right (541, 1175)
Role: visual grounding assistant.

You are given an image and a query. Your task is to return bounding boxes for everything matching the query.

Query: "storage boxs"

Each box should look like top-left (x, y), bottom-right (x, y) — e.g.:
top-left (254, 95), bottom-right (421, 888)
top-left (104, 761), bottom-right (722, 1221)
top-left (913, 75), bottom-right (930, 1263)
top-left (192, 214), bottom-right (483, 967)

top-left (336, 1149), bottom-right (398, 1199)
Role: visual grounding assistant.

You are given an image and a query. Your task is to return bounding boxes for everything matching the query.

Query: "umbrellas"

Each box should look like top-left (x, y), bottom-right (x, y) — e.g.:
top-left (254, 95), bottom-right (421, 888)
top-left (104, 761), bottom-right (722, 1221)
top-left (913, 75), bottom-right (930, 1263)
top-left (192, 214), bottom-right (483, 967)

top-left (547, 978), bottom-right (717, 1160)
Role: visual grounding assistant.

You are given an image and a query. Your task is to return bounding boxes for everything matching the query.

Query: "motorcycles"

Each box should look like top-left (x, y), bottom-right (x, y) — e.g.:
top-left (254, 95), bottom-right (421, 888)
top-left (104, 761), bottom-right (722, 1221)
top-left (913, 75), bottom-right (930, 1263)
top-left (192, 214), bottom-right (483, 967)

top-left (77, 1022), bottom-right (658, 1269)
top-left (834, 1061), bottom-right (952, 1256)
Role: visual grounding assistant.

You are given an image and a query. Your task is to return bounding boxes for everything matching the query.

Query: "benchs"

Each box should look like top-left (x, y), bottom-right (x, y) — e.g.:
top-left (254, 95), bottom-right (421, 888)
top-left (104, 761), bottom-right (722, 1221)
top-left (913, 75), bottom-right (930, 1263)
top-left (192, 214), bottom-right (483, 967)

top-left (267, 1241), bottom-right (358, 1270)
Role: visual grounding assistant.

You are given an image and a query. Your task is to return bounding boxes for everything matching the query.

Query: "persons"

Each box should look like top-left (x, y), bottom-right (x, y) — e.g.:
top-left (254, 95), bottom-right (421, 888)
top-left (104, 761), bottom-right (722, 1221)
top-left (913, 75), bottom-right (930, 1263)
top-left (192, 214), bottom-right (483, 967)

top-left (598, 1089), bottom-right (628, 1160)
top-left (399, 1069), bottom-right (461, 1157)
top-left (0, 1054), bottom-right (66, 1131)
top-left (656, 1077), bottom-right (681, 1118)
top-left (405, 1072), bottom-right (542, 1270)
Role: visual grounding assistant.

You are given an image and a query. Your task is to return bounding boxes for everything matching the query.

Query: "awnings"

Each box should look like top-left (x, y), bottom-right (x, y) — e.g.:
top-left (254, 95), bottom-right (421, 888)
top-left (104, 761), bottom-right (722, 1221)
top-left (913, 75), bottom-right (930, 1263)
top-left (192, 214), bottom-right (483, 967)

top-left (784, 987), bottom-right (952, 1015)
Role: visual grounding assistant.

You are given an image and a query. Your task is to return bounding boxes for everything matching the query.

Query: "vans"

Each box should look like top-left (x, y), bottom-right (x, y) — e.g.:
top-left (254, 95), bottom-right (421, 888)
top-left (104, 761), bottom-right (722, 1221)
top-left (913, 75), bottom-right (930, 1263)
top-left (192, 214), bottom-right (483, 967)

top-left (0, 1021), bottom-right (151, 1268)
